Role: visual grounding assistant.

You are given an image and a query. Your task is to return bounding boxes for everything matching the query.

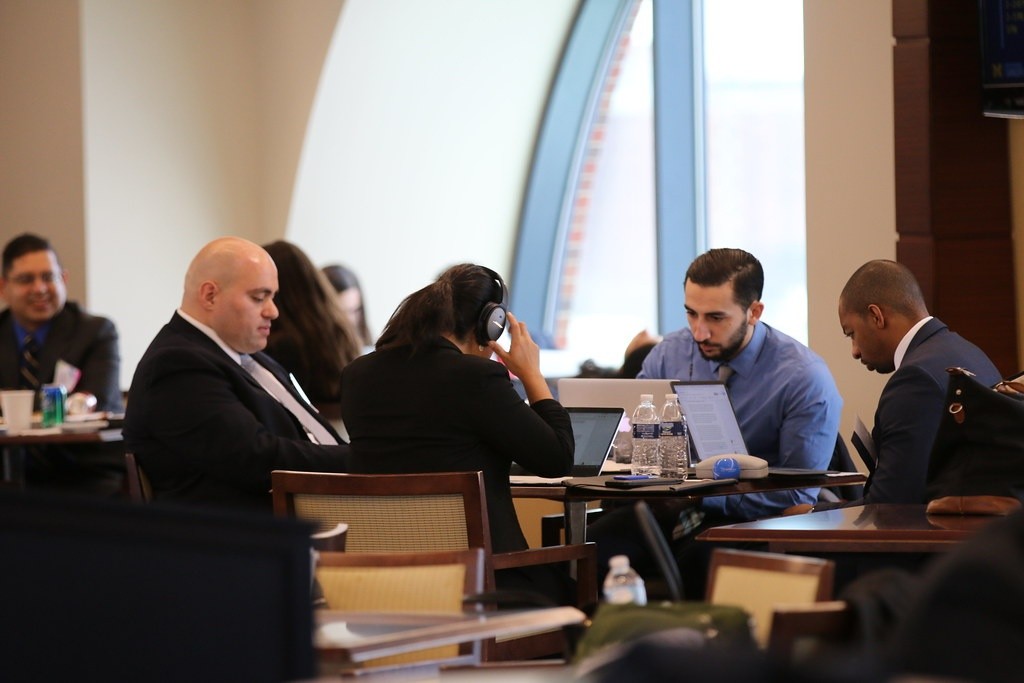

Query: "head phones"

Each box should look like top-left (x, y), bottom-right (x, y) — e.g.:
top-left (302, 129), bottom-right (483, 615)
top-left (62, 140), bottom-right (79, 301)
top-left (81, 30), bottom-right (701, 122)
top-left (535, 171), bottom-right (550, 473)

top-left (474, 266), bottom-right (508, 347)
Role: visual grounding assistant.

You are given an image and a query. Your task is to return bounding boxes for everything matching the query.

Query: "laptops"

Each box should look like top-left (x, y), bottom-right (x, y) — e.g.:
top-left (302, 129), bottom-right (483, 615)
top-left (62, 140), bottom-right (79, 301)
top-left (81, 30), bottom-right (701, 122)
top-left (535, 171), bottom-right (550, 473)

top-left (508, 377), bottom-right (840, 488)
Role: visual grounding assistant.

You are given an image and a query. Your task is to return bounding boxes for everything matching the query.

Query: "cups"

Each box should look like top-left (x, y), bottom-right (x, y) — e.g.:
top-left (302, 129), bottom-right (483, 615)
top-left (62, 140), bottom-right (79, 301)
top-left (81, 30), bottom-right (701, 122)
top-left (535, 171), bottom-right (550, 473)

top-left (0, 390), bottom-right (35, 429)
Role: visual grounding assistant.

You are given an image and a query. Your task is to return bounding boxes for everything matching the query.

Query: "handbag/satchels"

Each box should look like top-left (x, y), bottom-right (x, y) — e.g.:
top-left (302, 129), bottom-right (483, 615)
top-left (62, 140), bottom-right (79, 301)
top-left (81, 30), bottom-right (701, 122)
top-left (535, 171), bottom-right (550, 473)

top-left (923, 365), bottom-right (1024, 516)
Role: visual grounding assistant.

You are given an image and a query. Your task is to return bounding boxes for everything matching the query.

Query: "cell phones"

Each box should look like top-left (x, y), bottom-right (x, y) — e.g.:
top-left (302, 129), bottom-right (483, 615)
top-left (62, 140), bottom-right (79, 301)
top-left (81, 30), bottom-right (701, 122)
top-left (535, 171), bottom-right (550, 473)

top-left (614, 475), bottom-right (649, 480)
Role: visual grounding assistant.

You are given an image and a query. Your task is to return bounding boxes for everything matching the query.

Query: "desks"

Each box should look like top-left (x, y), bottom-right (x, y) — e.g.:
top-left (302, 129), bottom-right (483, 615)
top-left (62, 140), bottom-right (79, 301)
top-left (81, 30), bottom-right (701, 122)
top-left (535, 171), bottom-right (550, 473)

top-left (312, 603), bottom-right (581, 666)
top-left (695, 501), bottom-right (1002, 558)
top-left (0, 426), bottom-right (123, 446)
top-left (510, 469), bottom-right (870, 607)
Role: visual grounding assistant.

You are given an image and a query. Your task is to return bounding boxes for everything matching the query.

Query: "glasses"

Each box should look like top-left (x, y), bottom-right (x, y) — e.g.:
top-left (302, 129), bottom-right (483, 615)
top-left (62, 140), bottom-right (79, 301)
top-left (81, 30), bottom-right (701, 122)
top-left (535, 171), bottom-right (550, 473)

top-left (4, 271), bottom-right (62, 286)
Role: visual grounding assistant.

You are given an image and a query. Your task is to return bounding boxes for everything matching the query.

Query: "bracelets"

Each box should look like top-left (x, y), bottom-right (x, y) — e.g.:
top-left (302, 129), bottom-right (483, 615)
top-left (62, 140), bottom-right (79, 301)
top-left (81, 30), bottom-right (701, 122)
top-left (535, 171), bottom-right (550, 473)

top-left (807, 508), bottom-right (814, 513)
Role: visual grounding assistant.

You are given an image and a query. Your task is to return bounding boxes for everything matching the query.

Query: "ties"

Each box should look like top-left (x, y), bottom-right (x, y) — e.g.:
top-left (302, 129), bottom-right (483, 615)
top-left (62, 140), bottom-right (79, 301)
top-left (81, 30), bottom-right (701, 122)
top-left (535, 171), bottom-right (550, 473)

top-left (241, 353), bottom-right (338, 446)
top-left (22, 333), bottom-right (41, 389)
top-left (717, 364), bottom-right (733, 385)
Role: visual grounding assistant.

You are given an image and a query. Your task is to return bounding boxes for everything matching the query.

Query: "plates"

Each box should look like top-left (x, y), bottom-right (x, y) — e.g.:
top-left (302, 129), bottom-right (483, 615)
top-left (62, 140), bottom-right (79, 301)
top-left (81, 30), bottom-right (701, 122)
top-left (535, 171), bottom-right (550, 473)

top-left (63, 420), bottom-right (109, 433)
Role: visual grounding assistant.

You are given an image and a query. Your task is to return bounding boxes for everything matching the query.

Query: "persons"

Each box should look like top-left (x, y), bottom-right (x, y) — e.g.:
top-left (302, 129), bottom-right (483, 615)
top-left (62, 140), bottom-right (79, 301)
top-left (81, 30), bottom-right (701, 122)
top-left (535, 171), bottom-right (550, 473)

top-left (0, 235), bottom-right (122, 415)
top-left (783, 258), bottom-right (1002, 575)
top-left (586, 248), bottom-right (843, 598)
top-left (264, 242), bottom-right (371, 407)
top-left (575, 330), bottom-right (663, 379)
top-left (125, 237), bottom-right (354, 516)
top-left (338, 264), bottom-right (578, 606)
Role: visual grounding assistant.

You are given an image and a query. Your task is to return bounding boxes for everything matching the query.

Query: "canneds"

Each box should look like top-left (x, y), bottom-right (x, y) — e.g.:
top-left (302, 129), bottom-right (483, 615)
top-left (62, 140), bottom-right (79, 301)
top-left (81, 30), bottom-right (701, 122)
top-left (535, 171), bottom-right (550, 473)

top-left (65, 390), bottom-right (97, 416)
top-left (40, 383), bottom-right (66, 428)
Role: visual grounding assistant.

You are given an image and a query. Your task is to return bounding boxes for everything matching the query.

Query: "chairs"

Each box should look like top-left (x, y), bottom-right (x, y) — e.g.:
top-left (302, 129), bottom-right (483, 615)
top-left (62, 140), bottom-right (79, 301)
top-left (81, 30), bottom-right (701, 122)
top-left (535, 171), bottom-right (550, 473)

top-left (0, 428), bottom-right (834, 683)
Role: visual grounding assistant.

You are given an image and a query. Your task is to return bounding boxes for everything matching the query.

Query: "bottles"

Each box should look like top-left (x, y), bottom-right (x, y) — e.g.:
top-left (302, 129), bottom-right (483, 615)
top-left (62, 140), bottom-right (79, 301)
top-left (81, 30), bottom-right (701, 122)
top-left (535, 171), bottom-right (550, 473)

top-left (660, 391), bottom-right (688, 478)
top-left (630, 394), bottom-right (660, 478)
top-left (603, 555), bottom-right (647, 607)
